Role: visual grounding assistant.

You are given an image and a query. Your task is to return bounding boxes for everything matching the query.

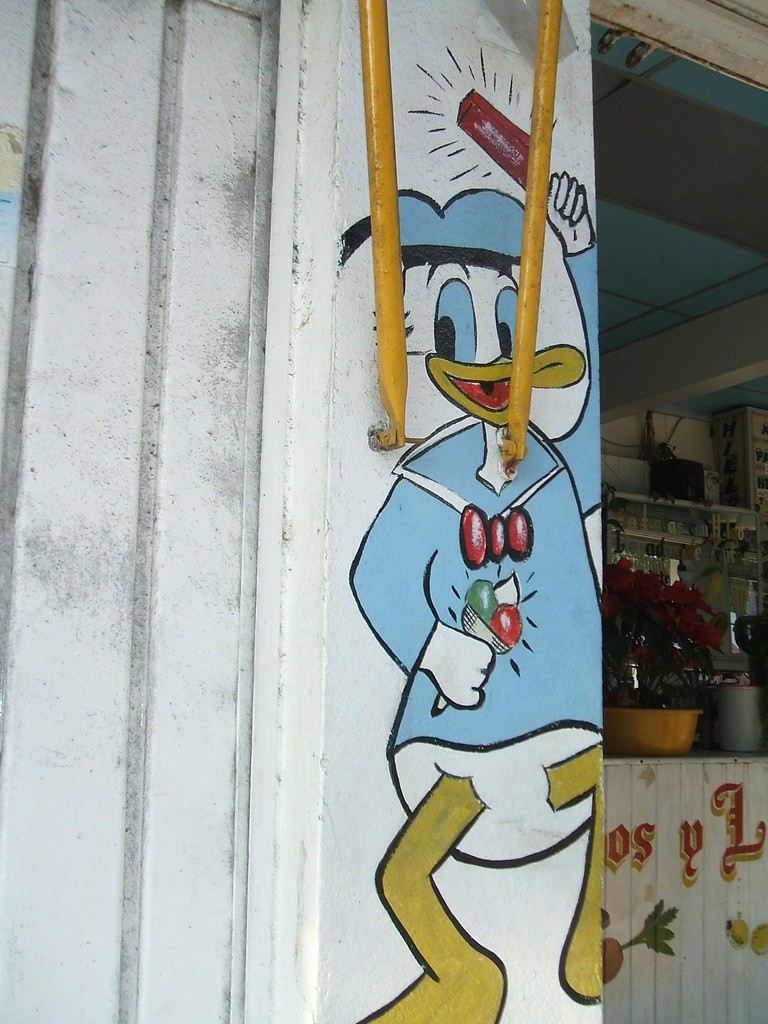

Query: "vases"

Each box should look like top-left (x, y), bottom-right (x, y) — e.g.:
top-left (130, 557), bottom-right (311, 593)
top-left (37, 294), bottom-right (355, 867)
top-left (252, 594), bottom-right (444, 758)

top-left (605, 707), bottom-right (702, 756)
top-left (717, 686), bottom-right (767, 752)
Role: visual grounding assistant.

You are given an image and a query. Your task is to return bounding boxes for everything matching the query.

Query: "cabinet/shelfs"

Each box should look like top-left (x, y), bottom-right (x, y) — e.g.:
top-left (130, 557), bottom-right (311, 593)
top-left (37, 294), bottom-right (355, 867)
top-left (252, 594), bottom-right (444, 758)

top-left (607, 405), bottom-right (768, 672)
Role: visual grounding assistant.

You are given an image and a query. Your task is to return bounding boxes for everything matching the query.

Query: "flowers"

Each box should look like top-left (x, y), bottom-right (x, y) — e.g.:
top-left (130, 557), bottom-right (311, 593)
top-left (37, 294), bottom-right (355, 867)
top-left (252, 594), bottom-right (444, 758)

top-left (601, 558), bottom-right (728, 707)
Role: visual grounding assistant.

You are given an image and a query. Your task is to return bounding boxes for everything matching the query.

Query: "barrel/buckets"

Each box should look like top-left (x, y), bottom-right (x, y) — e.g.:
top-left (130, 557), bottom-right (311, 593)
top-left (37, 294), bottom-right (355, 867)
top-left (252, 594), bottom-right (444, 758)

top-left (701, 674), bottom-right (768, 753)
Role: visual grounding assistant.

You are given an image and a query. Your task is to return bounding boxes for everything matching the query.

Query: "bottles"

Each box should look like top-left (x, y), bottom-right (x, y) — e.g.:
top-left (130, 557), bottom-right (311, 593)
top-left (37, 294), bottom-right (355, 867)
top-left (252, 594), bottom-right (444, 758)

top-left (610, 543), bottom-right (670, 585)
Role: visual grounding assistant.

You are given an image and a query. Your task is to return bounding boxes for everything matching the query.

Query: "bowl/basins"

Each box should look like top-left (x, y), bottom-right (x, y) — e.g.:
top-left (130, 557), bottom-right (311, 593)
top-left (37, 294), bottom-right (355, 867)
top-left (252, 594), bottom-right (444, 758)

top-left (603, 706), bottom-right (704, 758)
